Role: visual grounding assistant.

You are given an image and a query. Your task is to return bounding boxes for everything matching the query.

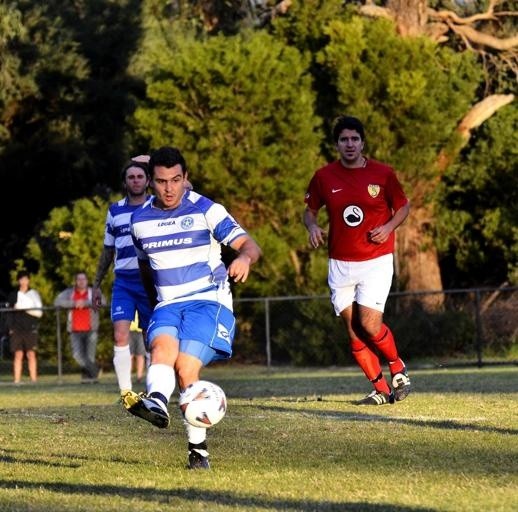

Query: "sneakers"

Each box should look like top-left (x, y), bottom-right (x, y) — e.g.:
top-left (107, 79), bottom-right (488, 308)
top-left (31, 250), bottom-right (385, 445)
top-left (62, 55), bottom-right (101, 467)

top-left (119, 388), bottom-right (171, 429)
top-left (187, 449), bottom-right (210, 473)
top-left (80, 370), bottom-right (102, 386)
top-left (353, 389), bottom-right (390, 407)
top-left (392, 369), bottom-right (410, 402)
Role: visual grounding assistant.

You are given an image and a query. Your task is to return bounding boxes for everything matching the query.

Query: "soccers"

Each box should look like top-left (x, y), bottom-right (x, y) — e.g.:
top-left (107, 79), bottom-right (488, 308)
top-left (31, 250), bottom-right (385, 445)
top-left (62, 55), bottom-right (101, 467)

top-left (179, 380), bottom-right (227, 428)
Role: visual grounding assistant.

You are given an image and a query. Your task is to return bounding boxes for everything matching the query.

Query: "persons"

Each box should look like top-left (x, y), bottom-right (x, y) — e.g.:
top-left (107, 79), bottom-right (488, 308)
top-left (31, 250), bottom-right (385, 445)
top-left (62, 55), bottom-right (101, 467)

top-left (53, 272), bottom-right (107, 384)
top-left (92, 154), bottom-right (193, 403)
top-left (4, 269), bottom-right (44, 384)
top-left (119, 146), bottom-right (261, 469)
top-left (127, 310), bottom-right (148, 387)
top-left (304, 117), bottom-right (411, 405)
